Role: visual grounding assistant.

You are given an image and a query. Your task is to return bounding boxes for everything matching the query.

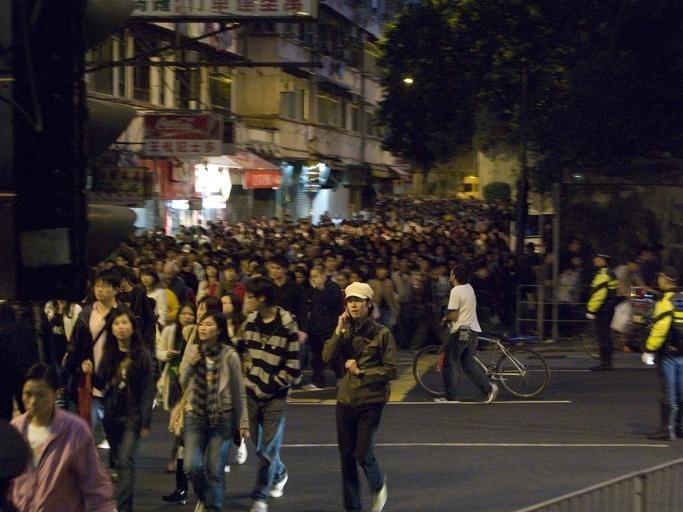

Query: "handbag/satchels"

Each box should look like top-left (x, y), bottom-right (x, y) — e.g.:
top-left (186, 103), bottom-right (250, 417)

top-left (168, 404), bottom-right (185, 438)
top-left (169, 383), bottom-right (182, 407)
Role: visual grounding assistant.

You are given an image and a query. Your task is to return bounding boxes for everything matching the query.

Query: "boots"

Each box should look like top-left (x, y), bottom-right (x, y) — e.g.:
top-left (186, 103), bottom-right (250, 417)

top-left (648, 400), bottom-right (678, 440)
top-left (590, 344), bottom-right (611, 371)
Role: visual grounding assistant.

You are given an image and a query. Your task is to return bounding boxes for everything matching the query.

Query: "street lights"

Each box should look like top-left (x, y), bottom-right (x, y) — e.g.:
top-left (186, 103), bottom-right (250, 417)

top-left (354, 6), bottom-right (378, 159)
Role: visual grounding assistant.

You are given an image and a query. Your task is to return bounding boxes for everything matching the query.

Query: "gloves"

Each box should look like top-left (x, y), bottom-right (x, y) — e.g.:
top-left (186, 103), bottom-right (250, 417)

top-left (641, 350), bottom-right (656, 366)
top-left (585, 312), bottom-right (596, 320)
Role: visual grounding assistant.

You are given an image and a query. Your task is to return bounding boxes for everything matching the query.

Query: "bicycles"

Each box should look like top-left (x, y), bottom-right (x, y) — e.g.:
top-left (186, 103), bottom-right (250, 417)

top-left (412, 317), bottom-right (551, 399)
top-left (580, 296), bottom-right (655, 362)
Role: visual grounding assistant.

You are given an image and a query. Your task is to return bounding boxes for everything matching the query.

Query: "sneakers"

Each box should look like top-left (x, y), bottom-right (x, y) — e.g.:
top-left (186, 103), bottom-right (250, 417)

top-left (163, 443), bottom-right (388, 512)
top-left (435, 396), bottom-right (460, 404)
top-left (485, 382), bottom-right (499, 404)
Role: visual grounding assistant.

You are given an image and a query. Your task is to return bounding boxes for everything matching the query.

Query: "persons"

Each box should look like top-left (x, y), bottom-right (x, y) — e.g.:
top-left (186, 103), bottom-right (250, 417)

top-left (238, 276), bottom-right (301, 512)
top-left (11, 367), bottom-right (119, 512)
top-left (320, 280), bottom-right (396, 512)
top-left (84, 306), bottom-right (154, 512)
top-left (544, 211), bottom-right (683, 441)
top-left (161, 294), bottom-right (221, 505)
top-left (178, 310), bottom-right (250, 512)
top-left (1, 178), bottom-right (543, 474)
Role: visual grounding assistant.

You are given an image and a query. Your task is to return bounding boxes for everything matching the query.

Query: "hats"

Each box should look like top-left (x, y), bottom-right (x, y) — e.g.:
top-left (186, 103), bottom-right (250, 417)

top-left (592, 249), bottom-right (613, 258)
top-left (344, 282), bottom-right (373, 301)
top-left (654, 266), bottom-right (680, 282)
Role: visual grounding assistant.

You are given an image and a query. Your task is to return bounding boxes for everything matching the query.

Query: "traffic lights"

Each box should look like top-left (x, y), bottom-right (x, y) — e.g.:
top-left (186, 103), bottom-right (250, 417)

top-left (0, 0), bottom-right (24, 299)
top-left (11, 0), bottom-right (137, 300)
